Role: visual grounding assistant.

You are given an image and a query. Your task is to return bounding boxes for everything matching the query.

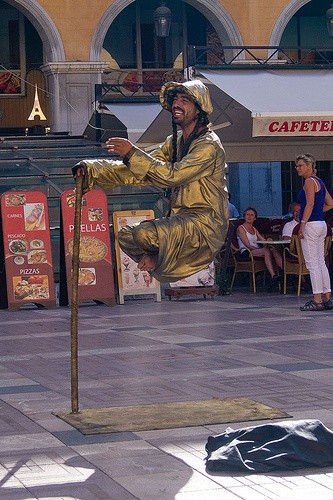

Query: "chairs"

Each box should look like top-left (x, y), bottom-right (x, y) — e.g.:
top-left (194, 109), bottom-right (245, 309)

top-left (281, 234), bottom-right (330, 297)
top-left (225, 236), bottom-right (270, 295)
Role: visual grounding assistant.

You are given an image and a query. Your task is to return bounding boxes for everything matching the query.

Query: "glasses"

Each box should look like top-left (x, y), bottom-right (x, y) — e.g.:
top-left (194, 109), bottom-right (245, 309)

top-left (295, 164), bottom-right (306, 168)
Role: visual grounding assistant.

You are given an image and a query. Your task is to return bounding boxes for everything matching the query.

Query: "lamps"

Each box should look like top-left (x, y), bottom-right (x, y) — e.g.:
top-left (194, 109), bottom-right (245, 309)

top-left (325, 7), bottom-right (333, 39)
top-left (149, 0), bottom-right (174, 38)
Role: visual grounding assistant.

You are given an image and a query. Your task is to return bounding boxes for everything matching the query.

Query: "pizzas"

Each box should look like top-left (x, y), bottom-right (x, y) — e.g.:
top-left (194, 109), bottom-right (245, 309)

top-left (121, 70), bottom-right (164, 93)
top-left (15, 284), bottom-right (49, 300)
top-left (78, 269), bottom-right (96, 285)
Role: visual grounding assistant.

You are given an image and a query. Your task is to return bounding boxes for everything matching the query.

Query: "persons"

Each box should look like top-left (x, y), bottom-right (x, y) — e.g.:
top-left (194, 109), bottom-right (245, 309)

top-left (296, 153), bottom-right (333, 311)
top-left (237, 206), bottom-right (283, 287)
top-left (72, 80), bottom-right (230, 283)
top-left (217, 193), bottom-right (241, 296)
top-left (282, 202), bottom-right (333, 294)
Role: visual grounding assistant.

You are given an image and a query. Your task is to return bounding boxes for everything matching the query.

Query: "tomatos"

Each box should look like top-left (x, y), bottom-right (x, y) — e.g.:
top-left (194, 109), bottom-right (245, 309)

top-left (28, 276), bottom-right (43, 284)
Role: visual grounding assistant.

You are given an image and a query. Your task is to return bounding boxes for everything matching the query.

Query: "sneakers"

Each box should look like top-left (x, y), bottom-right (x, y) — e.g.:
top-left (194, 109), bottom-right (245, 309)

top-left (299, 299), bottom-right (325, 311)
top-left (322, 299), bottom-right (333, 310)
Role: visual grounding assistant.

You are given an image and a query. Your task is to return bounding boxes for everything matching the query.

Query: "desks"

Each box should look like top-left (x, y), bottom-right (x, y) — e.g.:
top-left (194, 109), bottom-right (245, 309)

top-left (254, 239), bottom-right (292, 294)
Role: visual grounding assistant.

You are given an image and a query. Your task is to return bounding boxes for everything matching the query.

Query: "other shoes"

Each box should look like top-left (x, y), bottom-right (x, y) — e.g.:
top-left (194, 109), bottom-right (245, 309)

top-left (272, 275), bottom-right (283, 286)
top-left (279, 268), bottom-right (284, 275)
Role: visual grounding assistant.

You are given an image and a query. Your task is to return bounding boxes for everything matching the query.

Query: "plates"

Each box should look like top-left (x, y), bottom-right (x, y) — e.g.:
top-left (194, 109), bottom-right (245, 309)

top-left (30, 239), bottom-right (44, 249)
top-left (9, 240), bottom-right (27, 255)
top-left (66, 236), bottom-right (107, 263)
top-left (27, 250), bottom-right (47, 263)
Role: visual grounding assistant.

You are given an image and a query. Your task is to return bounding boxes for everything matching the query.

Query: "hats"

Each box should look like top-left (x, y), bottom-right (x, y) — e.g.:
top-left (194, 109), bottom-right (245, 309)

top-left (159, 79), bottom-right (213, 114)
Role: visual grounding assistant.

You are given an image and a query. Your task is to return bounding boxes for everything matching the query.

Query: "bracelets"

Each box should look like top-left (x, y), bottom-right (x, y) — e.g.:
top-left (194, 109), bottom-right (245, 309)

top-left (301, 220), bottom-right (308, 224)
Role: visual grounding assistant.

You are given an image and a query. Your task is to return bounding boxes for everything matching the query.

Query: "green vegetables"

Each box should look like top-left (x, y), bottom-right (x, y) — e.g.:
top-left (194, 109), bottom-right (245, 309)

top-left (10, 241), bottom-right (26, 252)
